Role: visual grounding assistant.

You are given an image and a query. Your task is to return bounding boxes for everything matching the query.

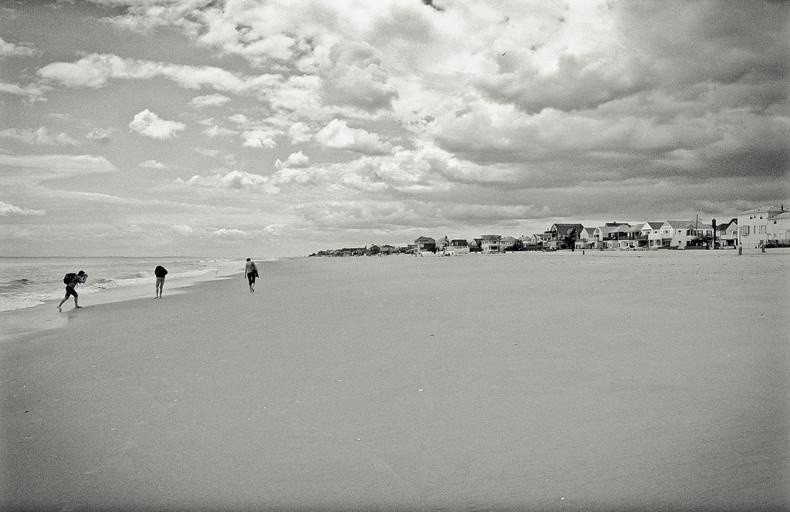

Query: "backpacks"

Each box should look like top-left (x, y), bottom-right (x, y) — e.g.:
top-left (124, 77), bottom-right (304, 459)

top-left (64, 273), bottom-right (77, 284)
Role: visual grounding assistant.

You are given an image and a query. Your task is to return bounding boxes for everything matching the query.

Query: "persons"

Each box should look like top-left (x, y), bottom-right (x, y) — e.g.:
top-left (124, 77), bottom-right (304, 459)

top-left (57, 271), bottom-right (88, 310)
top-left (155, 266), bottom-right (168, 298)
top-left (244, 258), bottom-right (259, 294)
